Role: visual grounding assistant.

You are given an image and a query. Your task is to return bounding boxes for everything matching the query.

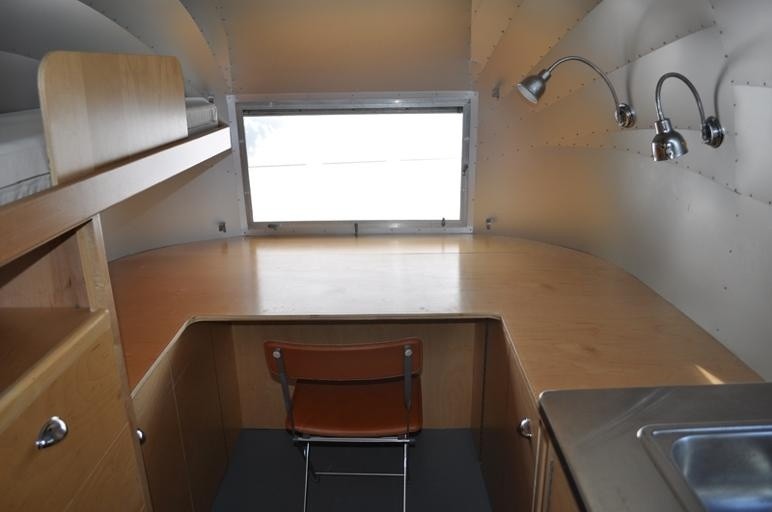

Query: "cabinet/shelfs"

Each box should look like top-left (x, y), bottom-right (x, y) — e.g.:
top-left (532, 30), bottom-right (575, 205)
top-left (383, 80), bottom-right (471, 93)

top-left (484, 321), bottom-right (546, 512)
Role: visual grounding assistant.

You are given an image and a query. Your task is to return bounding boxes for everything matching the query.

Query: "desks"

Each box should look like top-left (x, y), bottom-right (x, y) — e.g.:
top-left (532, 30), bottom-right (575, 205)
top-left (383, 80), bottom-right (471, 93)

top-left (109, 234), bottom-right (772, 512)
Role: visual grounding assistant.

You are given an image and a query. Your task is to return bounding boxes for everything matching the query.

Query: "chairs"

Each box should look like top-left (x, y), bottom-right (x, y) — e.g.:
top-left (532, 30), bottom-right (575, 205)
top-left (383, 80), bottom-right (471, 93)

top-left (262, 338), bottom-right (423, 512)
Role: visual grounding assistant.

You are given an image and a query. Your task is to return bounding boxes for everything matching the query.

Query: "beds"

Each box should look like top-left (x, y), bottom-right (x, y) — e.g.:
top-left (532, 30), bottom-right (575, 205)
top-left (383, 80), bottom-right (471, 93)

top-left (0, 49), bottom-right (231, 512)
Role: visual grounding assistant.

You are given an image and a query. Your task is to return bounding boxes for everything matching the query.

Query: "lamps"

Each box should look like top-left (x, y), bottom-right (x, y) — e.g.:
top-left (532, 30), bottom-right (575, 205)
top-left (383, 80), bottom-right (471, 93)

top-left (652, 72), bottom-right (724, 161)
top-left (517, 56), bottom-right (636, 127)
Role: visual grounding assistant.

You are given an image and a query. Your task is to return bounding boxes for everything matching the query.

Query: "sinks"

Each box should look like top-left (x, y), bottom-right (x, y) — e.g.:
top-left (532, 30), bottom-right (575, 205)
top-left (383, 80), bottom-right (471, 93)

top-left (538, 382), bottom-right (772, 512)
